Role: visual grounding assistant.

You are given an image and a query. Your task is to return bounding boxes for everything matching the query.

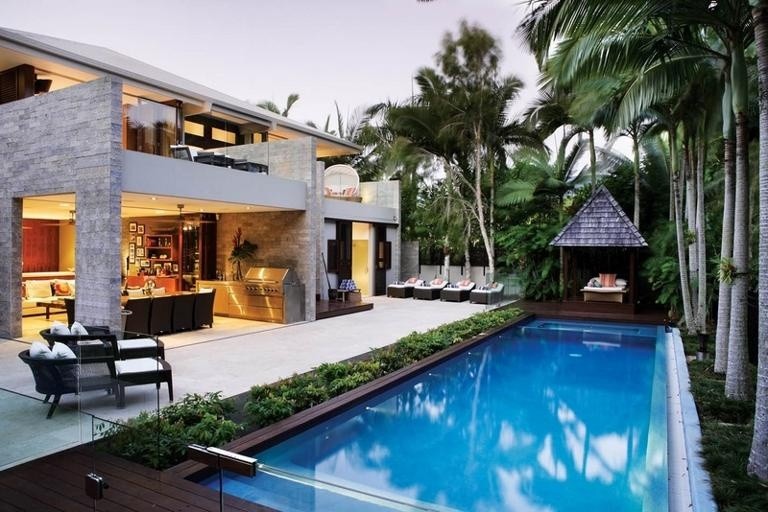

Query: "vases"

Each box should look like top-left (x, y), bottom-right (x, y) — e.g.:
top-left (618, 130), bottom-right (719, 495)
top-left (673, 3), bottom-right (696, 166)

top-left (234, 260), bottom-right (245, 281)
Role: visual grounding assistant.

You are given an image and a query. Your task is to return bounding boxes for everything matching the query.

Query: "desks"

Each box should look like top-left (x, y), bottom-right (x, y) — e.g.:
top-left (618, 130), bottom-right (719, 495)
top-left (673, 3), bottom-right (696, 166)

top-left (35, 300), bottom-right (66, 319)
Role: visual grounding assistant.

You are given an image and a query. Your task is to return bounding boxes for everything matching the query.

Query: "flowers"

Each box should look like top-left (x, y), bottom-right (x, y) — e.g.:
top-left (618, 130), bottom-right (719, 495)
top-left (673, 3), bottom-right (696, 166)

top-left (227, 227), bottom-right (258, 264)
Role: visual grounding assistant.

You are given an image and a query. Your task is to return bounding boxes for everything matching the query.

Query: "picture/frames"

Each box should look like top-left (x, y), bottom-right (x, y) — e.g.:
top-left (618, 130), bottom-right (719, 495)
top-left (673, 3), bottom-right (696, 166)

top-left (153, 262), bottom-right (179, 273)
top-left (128, 221), bottom-right (151, 267)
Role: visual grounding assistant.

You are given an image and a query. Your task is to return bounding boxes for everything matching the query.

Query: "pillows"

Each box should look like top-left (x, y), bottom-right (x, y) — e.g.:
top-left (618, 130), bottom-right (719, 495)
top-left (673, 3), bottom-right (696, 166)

top-left (50, 320), bottom-right (70, 335)
top-left (52, 341), bottom-right (81, 373)
top-left (599, 273), bottom-right (617, 287)
top-left (50, 280), bottom-right (72, 296)
top-left (589, 276), bottom-right (628, 285)
top-left (29, 340), bottom-right (51, 359)
top-left (587, 344), bottom-right (609, 352)
top-left (70, 321), bottom-right (89, 335)
top-left (25, 279), bottom-right (53, 300)
top-left (587, 278), bottom-right (602, 288)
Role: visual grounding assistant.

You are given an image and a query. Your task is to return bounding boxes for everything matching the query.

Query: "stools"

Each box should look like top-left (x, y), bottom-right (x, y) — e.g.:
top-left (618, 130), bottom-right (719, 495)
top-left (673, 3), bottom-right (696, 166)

top-left (117, 336), bottom-right (165, 360)
top-left (114, 356), bottom-right (174, 409)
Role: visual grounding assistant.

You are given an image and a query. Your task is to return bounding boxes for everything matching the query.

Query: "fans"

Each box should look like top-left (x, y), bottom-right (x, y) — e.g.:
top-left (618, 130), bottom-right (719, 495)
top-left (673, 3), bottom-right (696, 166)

top-left (151, 204), bottom-right (218, 225)
top-left (38, 209), bottom-right (75, 226)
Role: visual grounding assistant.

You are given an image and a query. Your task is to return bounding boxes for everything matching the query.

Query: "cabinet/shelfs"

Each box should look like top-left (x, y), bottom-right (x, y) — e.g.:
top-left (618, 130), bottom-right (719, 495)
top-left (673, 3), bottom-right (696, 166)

top-left (127, 276), bottom-right (179, 289)
top-left (179, 214), bottom-right (216, 291)
top-left (144, 234), bottom-right (176, 263)
top-left (195, 278), bottom-right (239, 317)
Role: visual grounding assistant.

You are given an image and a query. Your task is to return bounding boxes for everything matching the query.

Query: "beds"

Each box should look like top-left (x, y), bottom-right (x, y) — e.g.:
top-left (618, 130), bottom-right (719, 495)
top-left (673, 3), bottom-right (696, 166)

top-left (578, 272), bottom-right (630, 304)
top-left (581, 330), bottom-right (622, 350)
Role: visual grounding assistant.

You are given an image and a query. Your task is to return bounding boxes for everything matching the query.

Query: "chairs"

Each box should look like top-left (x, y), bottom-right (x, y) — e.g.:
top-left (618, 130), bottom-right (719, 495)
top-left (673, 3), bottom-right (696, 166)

top-left (385, 277), bottom-right (504, 305)
top-left (18, 348), bottom-right (122, 419)
top-left (167, 143), bottom-right (270, 176)
top-left (64, 286), bottom-right (217, 337)
top-left (39, 325), bottom-right (120, 360)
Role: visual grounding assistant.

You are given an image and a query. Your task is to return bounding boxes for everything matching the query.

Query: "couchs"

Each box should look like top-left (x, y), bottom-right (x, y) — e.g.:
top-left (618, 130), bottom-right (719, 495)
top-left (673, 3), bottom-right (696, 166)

top-left (21, 278), bottom-right (75, 317)
